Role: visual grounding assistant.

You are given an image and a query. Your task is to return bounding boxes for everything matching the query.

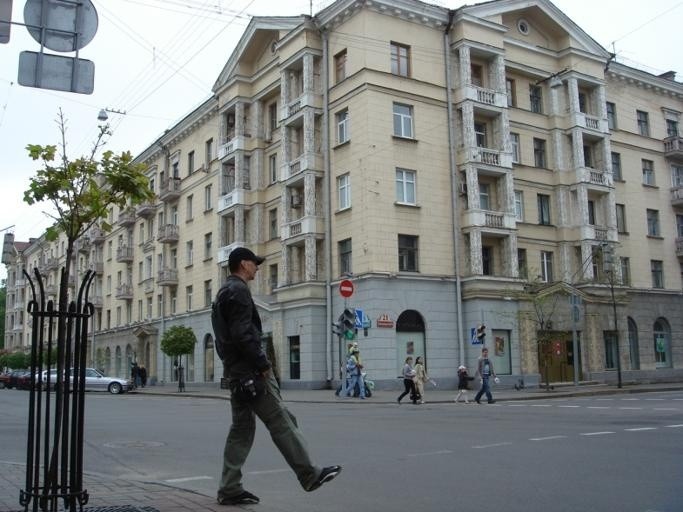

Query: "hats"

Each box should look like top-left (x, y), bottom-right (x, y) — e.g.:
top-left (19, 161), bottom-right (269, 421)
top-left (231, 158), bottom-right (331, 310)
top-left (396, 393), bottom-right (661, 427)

top-left (227, 247), bottom-right (266, 266)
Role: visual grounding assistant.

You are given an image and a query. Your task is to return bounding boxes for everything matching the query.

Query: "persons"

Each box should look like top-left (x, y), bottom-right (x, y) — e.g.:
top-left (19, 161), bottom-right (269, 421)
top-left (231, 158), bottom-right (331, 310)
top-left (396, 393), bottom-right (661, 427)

top-left (395, 356), bottom-right (436, 406)
top-left (210, 247), bottom-right (343, 505)
top-left (454, 346), bottom-right (499, 404)
top-left (131, 362), bottom-right (147, 388)
top-left (335, 343), bottom-right (373, 399)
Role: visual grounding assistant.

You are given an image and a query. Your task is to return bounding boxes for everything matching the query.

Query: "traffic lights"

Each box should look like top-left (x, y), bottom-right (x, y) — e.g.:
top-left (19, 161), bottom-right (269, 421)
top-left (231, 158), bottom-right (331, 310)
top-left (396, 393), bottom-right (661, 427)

top-left (329, 308), bottom-right (356, 341)
top-left (477, 325), bottom-right (486, 340)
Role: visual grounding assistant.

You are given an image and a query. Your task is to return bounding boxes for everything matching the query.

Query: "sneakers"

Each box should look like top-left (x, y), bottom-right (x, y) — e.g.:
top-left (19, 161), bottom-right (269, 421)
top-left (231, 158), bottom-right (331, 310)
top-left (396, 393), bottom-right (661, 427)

top-left (453, 400), bottom-right (497, 405)
top-left (217, 490), bottom-right (260, 505)
top-left (306, 465), bottom-right (342, 492)
top-left (397, 398), bottom-right (425, 406)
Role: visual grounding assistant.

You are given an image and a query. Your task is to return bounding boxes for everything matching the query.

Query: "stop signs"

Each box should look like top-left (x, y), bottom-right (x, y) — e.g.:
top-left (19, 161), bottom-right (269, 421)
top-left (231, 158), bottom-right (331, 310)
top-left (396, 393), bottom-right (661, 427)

top-left (340, 279), bottom-right (354, 298)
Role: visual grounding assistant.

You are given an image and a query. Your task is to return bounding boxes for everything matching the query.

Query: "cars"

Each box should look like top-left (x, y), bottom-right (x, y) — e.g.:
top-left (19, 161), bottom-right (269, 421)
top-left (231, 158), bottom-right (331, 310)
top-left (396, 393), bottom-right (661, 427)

top-left (0, 367), bottom-right (135, 394)
